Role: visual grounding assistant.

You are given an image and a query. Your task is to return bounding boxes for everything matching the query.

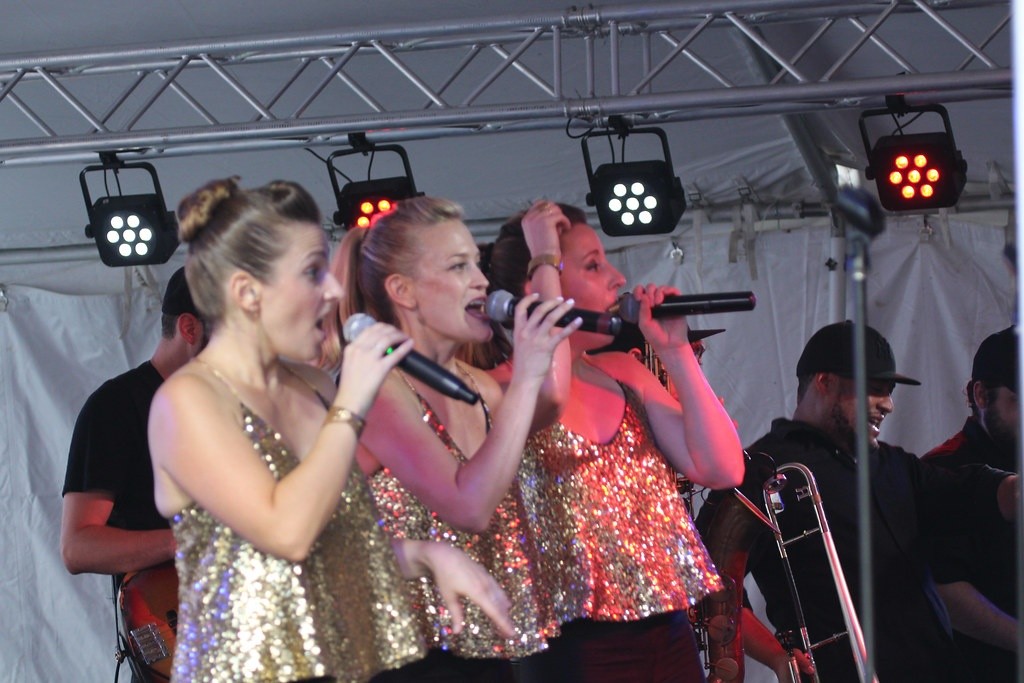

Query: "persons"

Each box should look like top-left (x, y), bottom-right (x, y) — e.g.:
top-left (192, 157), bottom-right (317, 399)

top-left (456, 198), bottom-right (746, 683)
top-left (313, 197), bottom-right (582, 683)
top-left (692, 319), bottom-right (1024, 683)
top-left (149, 175), bottom-right (517, 683)
top-left (919, 325), bottom-right (1024, 683)
top-left (60, 267), bottom-right (208, 682)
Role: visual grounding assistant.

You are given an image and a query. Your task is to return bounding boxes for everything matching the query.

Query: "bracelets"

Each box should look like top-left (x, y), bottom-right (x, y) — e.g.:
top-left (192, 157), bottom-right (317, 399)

top-left (323, 407), bottom-right (366, 440)
top-left (527, 254), bottom-right (563, 281)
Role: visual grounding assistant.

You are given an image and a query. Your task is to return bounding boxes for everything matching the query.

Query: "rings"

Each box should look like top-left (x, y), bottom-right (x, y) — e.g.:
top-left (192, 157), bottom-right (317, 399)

top-left (545, 208), bottom-right (553, 215)
top-left (538, 202), bottom-right (550, 211)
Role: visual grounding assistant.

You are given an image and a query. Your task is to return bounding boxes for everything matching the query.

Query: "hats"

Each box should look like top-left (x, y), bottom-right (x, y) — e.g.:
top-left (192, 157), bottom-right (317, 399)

top-left (797, 320), bottom-right (921, 385)
top-left (972, 324), bottom-right (1019, 386)
top-left (162, 267), bottom-right (197, 314)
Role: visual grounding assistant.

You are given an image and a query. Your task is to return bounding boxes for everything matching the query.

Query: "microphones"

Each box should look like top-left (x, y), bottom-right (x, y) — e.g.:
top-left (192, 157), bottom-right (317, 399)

top-left (486, 289), bottom-right (620, 335)
top-left (618, 290), bottom-right (754, 324)
top-left (341, 314), bottom-right (476, 405)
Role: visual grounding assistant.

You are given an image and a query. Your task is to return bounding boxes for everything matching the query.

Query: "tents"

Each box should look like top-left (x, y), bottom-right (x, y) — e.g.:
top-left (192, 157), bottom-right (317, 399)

top-left (0, 0), bottom-right (1024, 683)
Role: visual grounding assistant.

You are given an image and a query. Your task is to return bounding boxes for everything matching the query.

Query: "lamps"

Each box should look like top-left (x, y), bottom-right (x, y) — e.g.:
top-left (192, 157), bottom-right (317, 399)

top-left (859, 95), bottom-right (968, 212)
top-left (581, 115), bottom-right (686, 237)
top-left (80, 151), bottom-right (181, 267)
top-left (326, 133), bottom-right (425, 231)
